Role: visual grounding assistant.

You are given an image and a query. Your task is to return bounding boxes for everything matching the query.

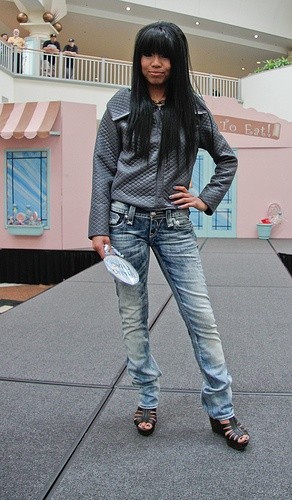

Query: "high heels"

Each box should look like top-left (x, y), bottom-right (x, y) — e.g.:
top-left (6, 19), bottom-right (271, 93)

top-left (209, 415), bottom-right (251, 450)
top-left (133, 406), bottom-right (157, 436)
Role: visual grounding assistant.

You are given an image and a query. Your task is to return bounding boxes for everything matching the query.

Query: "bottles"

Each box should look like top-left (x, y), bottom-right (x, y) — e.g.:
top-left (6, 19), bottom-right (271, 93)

top-left (25, 205), bottom-right (33, 225)
top-left (13, 204), bottom-right (19, 222)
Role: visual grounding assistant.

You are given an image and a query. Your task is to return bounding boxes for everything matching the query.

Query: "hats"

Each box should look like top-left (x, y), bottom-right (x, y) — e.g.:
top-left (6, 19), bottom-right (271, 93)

top-left (50, 34), bottom-right (56, 38)
top-left (68, 39), bottom-right (74, 42)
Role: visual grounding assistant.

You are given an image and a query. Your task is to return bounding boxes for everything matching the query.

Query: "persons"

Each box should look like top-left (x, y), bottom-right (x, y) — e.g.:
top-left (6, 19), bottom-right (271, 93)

top-left (88, 22), bottom-right (250, 449)
top-left (63, 39), bottom-right (79, 79)
top-left (42, 34), bottom-right (62, 77)
top-left (0, 33), bottom-right (7, 57)
top-left (8, 28), bottom-right (26, 74)
